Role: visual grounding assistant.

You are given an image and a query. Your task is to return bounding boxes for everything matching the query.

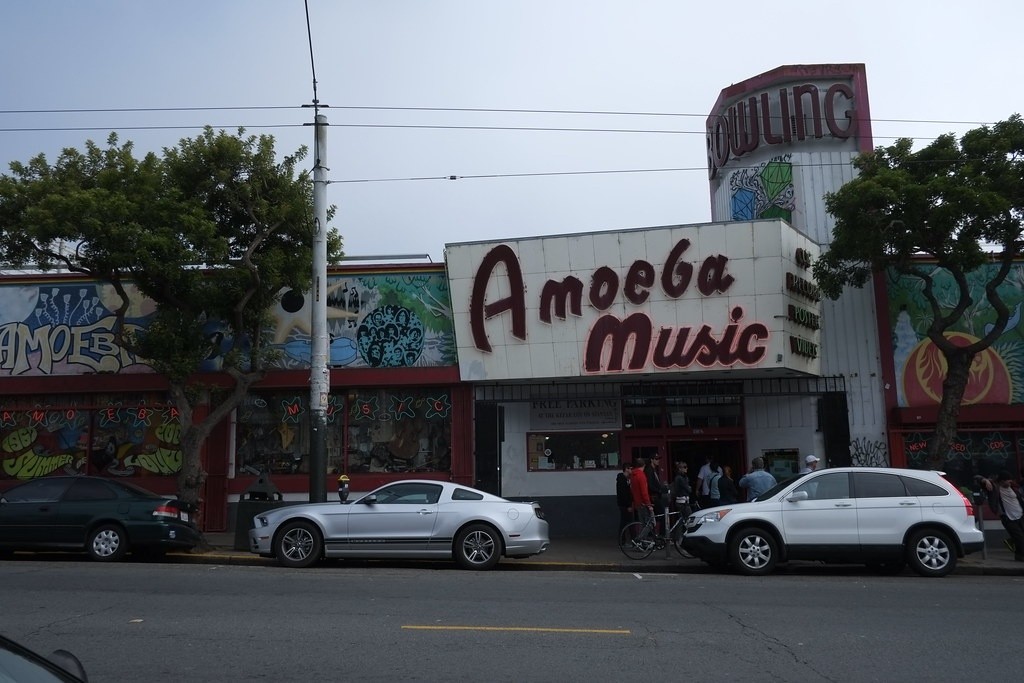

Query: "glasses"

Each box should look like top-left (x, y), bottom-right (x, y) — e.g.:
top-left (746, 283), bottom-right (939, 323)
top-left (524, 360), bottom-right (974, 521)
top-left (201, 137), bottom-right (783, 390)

top-left (680, 466), bottom-right (688, 469)
top-left (812, 461), bottom-right (817, 463)
top-left (627, 468), bottom-right (633, 470)
top-left (652, 458), bottom-right (659, 460)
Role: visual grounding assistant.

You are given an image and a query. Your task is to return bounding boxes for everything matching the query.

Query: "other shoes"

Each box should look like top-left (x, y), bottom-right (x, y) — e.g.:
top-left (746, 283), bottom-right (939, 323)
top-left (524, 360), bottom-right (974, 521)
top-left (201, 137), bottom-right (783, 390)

top-left (619, 544), bottom-right (625, 548)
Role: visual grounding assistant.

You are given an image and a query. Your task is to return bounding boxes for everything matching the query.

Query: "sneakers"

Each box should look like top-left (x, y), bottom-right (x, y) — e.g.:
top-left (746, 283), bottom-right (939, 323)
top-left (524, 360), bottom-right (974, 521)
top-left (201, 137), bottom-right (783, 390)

top-left (632, 538), bottom-right (644, 551)
top-left (648, 540), bottom-right (656, 547)
top-left (1004, 539), bottom-right (1015, 551)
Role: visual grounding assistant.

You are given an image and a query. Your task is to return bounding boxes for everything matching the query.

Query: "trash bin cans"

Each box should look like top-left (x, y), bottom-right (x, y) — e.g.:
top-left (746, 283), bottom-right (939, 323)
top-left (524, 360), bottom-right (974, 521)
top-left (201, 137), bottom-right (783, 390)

top-left (234, 473), bottom-right (285, 551)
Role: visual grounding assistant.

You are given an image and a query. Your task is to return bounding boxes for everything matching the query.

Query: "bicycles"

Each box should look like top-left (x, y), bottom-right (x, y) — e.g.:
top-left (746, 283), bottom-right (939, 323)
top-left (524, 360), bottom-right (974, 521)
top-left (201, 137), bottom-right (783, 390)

top-left (619, 495), bottom-right (701, 560)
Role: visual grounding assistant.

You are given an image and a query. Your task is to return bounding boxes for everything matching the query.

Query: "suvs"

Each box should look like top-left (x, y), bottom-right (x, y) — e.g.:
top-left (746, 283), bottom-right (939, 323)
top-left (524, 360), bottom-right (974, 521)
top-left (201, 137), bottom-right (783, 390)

top-left (680, 466), bottom-right (985, 577)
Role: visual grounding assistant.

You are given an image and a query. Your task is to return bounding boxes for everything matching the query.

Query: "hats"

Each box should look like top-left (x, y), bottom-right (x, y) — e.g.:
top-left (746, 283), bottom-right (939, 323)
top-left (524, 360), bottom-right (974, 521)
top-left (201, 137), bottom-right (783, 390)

top-left (1000, 470), bottom-right (1013, 480)
top-left (805, 455), bottom-right (820, 463)
top-left (648, 452), bottom-right (662, 458)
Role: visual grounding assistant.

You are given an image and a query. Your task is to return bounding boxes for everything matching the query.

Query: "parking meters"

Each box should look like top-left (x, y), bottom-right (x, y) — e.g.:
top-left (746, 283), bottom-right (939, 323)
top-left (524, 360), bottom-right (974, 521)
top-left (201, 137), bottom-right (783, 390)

top-left (661, 481), bottom-right (672, 558)
top-left (337, 474), bottom-right (351, 504)
top-left (972, 483), bottom-right (989, 561)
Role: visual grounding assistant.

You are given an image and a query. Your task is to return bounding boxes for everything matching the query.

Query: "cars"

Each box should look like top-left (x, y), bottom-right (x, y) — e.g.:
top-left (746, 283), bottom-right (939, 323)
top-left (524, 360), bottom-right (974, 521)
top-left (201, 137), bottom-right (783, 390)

top-left (248, 478), bottom-right (551, 569)
top-left (0, 475), bottom-right (198, 563)
top-left (1, 632), bottom-right (88, 683)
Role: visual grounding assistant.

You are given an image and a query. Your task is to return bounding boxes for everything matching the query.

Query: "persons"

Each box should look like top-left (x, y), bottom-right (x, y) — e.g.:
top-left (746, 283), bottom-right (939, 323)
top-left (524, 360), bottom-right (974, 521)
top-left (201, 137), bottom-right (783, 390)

top-left (975, 471), bottom-right (1024, 563)
top-left (615, 452), bottom-right (821, 552)
